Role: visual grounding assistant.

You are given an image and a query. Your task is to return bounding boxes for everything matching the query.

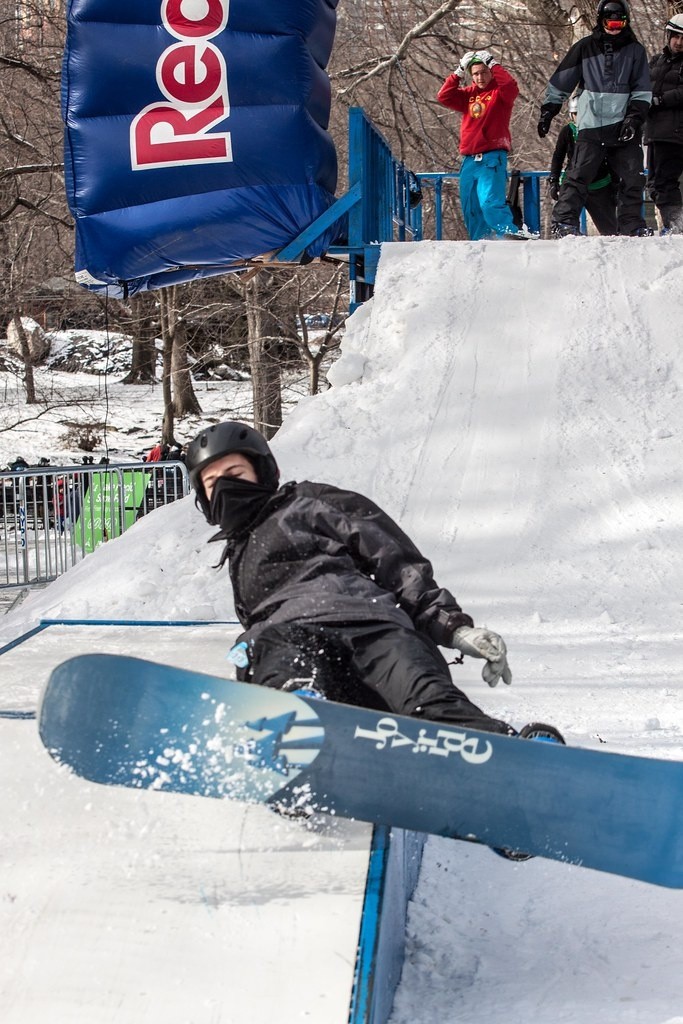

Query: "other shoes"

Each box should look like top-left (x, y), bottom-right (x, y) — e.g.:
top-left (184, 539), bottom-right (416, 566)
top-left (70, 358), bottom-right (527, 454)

top-left (555, 223), bottom-right (580, 238)
top-left (270, 678), bottom-right (325, 822)
top-left (633, 227), bottom-right (654, 237)
top-left (661, 227), bottom-right (671, 236)
top-left (499, 724), bottom-right (566, 861)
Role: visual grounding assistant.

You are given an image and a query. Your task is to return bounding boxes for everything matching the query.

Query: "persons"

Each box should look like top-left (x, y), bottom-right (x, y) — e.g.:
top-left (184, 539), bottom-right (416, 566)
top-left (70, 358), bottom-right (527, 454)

top-left (8, 457), bottom-right (32, 514)
top-left (538, 0), bottom-right (683, 239)
top-left (51, 480), bottom-right (71, 536)
top-left (436, 51), bottom-right (519, 241)
top-left (188, 423), bottom-right (569, 864)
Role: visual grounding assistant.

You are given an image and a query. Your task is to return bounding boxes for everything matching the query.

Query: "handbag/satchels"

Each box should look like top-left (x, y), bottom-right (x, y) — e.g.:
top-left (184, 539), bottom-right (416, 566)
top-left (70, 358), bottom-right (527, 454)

top-left (404, 170), bottom-right (423, 210)
top-left (505, 170), bottom-right (523, 230)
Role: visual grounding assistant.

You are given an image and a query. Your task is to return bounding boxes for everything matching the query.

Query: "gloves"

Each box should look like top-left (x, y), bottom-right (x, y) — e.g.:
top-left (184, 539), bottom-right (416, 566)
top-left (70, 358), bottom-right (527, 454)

top-left (451, 626), bottom-right (513, 688)
top-left (617, 118), bottom-right (638, 143)
top-left (475, 50), bottom-right (500, 70)
top-left (551, 181), bottom-right (560, 200)
top-left (649, 94), bottom-right (662, 110)
top-left (537, 110), bottom-right (553, 138)
top-left (454, 51), bottom-right (475, 78)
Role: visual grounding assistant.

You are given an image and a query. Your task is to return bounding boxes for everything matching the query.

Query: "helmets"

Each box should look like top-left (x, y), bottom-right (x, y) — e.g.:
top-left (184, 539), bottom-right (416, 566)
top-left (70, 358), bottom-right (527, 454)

top-left (186, 422), bottom-right (278, 523)
top-left (569, 95), bottom-right (581, 121)
top-left (665, 13), bottom-right (683, 33)
top-left (468, 53), bottom-right (485, 75)
top-left (601, 1), bottom-right (627, 20)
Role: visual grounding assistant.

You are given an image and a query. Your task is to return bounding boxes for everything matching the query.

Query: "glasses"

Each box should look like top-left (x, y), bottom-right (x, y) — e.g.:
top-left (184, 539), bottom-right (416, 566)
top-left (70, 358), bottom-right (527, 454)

top-left (601, 17), bottom-right (628, 30)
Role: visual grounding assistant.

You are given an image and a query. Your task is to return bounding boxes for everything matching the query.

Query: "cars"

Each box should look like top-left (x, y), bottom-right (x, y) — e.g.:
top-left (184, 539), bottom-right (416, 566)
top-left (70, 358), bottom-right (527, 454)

top-left (296, 313), bottom-right (347, 327)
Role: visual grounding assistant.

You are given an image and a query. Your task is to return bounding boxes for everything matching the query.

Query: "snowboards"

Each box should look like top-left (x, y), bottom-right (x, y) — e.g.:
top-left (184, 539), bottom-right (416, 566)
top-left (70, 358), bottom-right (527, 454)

top-left (36, 651), bottom-right (682, 891)
